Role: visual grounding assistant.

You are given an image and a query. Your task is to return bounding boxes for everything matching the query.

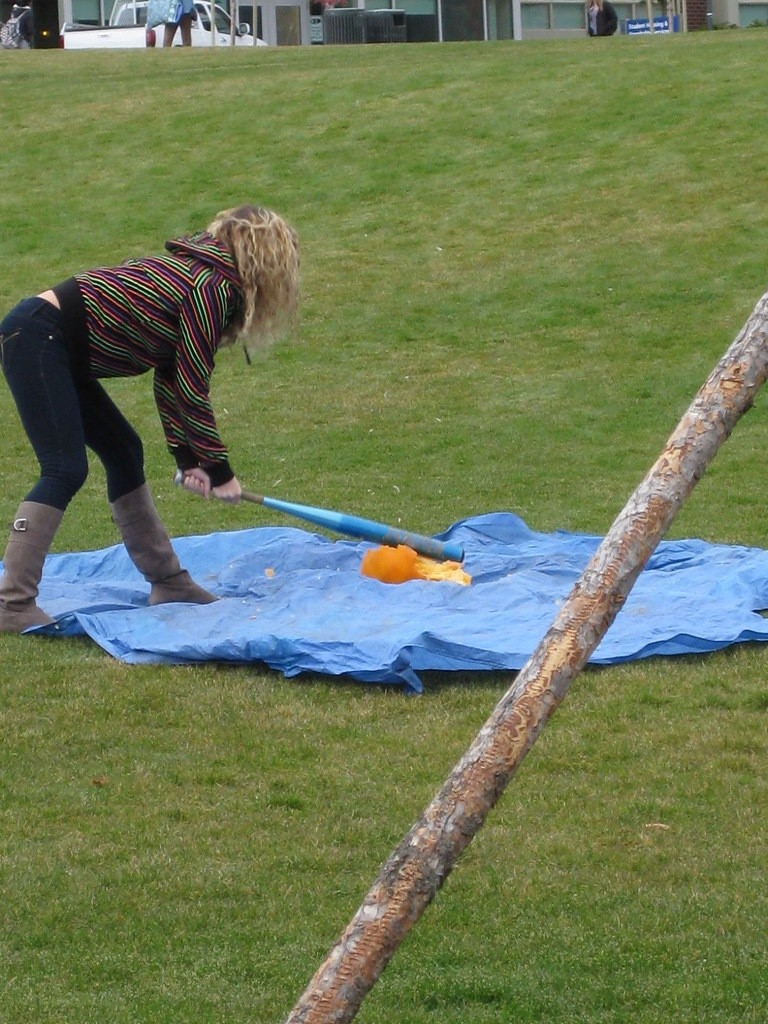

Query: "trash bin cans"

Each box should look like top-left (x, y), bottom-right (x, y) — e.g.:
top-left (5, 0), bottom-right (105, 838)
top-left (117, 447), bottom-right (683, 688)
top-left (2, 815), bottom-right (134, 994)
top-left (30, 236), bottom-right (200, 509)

top-left (366, 9), bottom-right (407, 43)
top-left (323, 8), bottom-right (367, 45)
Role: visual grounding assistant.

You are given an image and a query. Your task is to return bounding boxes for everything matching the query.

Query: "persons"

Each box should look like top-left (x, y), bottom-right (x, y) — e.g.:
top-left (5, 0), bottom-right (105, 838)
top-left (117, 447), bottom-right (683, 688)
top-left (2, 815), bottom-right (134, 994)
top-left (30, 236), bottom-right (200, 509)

top-left (163, 0), bottom-right (197, 47)
top-left (0, 203), bottom-right (301, 631)
top-left (586, 0), bottom-right (618, 37)
top-left (10, 0), bottom-right (37, 49)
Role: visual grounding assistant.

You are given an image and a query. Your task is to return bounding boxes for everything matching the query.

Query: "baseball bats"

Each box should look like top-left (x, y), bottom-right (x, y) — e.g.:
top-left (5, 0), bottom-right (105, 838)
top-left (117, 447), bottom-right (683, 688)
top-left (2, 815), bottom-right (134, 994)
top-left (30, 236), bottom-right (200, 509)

top-left (172, 469), bottom-right (465, 562)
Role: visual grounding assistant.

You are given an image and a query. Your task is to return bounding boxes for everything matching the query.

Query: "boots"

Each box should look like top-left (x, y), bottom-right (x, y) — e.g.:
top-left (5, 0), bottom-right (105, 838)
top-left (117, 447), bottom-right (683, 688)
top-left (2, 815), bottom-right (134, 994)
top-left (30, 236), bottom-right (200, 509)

top-left (109, 481), bottom-right (220, 606)
top-left (0, 501), bottom-right (66, 629)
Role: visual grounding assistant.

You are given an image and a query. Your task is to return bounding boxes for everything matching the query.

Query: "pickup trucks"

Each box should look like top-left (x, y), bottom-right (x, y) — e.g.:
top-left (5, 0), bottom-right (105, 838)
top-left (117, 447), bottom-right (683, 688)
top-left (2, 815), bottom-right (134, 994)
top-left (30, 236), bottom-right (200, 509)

top-left (58, 1), bottom-right (267, 48)
